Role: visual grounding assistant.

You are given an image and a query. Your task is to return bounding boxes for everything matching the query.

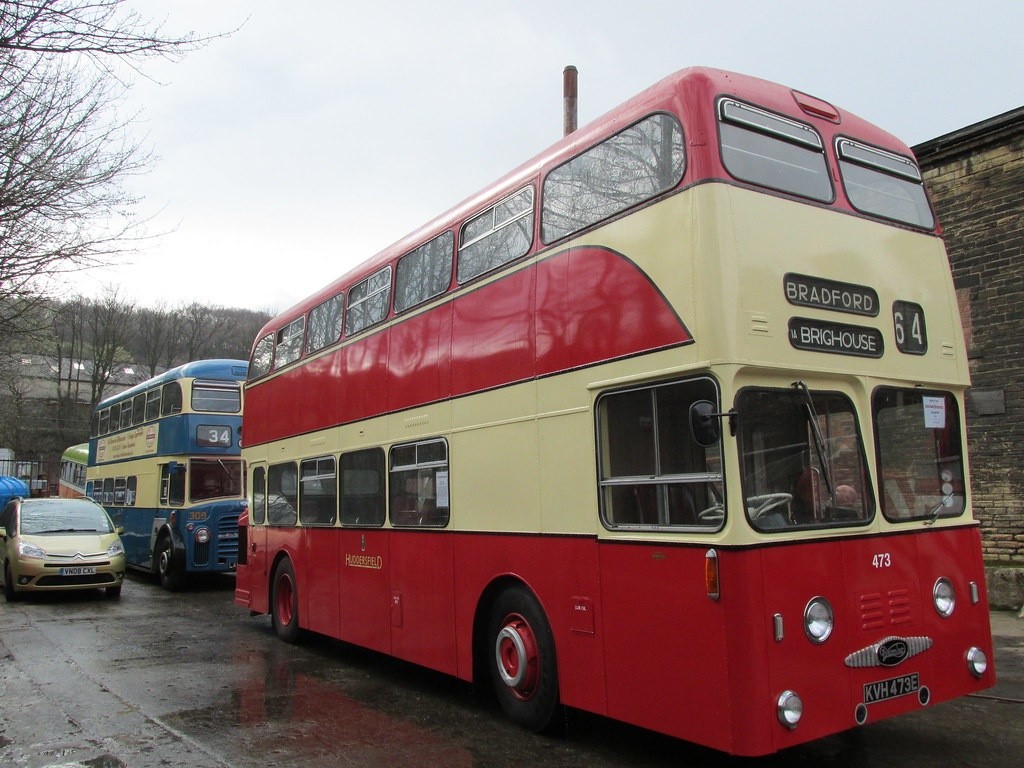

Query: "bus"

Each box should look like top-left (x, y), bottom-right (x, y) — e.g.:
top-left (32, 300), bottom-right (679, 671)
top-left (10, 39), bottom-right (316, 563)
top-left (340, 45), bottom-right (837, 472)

top-left (235, 65), bottom-right (997, 760)
top-left (57, 442), bottom-right (89, 500)
top-left (84, 359), bottom-right (250, 592)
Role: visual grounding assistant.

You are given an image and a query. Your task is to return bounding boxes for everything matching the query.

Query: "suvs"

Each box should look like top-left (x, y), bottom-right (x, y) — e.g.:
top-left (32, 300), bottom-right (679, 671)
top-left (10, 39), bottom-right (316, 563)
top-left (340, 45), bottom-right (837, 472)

top-left (0, 496), bottom-right (125, 601)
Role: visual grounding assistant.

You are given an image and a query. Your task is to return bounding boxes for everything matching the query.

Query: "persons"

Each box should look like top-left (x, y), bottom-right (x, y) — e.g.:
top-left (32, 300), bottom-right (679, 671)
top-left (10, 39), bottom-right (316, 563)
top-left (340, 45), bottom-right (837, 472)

top-left (824, 485), bottom-right (859, 521)
